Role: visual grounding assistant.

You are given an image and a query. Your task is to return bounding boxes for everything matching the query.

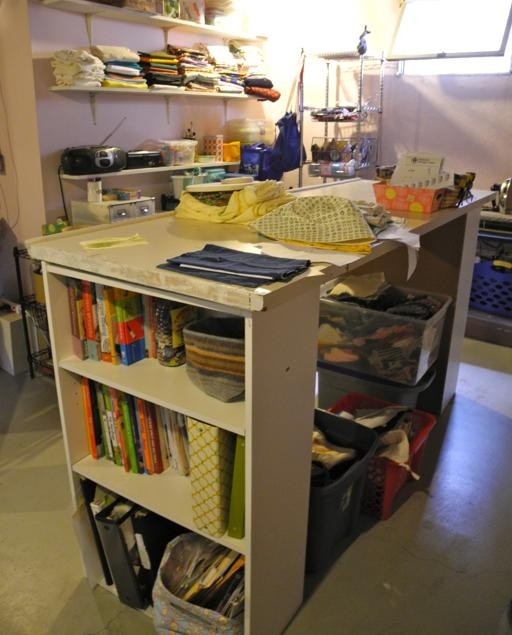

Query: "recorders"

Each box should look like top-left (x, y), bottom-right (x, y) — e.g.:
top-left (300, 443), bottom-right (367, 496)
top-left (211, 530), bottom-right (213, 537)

top-left (59, 143), bottom-right (127, 175)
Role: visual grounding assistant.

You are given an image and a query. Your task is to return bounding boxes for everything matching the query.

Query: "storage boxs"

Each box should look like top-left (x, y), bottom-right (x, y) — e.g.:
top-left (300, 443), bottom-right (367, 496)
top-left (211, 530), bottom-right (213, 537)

top-left (318, 283), bottom-right (450, 386)
top-left (312, 407), bottom-right (379, 552)
top-left (316, 363), bottom-right (437, 410)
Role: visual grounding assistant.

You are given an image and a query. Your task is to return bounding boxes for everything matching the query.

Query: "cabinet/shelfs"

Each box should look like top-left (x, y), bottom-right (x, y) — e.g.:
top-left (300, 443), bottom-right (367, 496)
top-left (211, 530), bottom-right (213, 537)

top-left (25, 0), bottom-right (281, 244)
top-left (23, 177), bottom-right (502, 634)
top-left (295, 48), bottom-right (385, 189)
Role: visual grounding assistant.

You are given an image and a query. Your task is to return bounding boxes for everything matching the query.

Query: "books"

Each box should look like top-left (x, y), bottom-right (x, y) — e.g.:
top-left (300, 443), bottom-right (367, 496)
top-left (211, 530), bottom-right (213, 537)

top-left (390, 155), bottom-right (444, 189)
top-left (81, 479), bottom-right (245, 618)
top-left (67, 280), bottom-right (197, 367)
top-left (81, 377), bottom-right (190, 475)
top-left (403, 169), bottom-right (456, 191)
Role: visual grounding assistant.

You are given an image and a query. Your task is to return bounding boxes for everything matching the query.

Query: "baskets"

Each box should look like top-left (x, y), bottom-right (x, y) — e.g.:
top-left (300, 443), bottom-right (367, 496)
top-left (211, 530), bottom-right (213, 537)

top-left (468, 255), bottom-right (512, 316)
top-left (439, 187), bottom-right (460, 209)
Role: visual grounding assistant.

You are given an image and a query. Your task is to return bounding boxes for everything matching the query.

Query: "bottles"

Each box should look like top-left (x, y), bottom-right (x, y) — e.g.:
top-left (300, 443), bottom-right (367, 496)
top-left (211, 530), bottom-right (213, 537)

top-left (87, 179), bottom-right (102, 203)
